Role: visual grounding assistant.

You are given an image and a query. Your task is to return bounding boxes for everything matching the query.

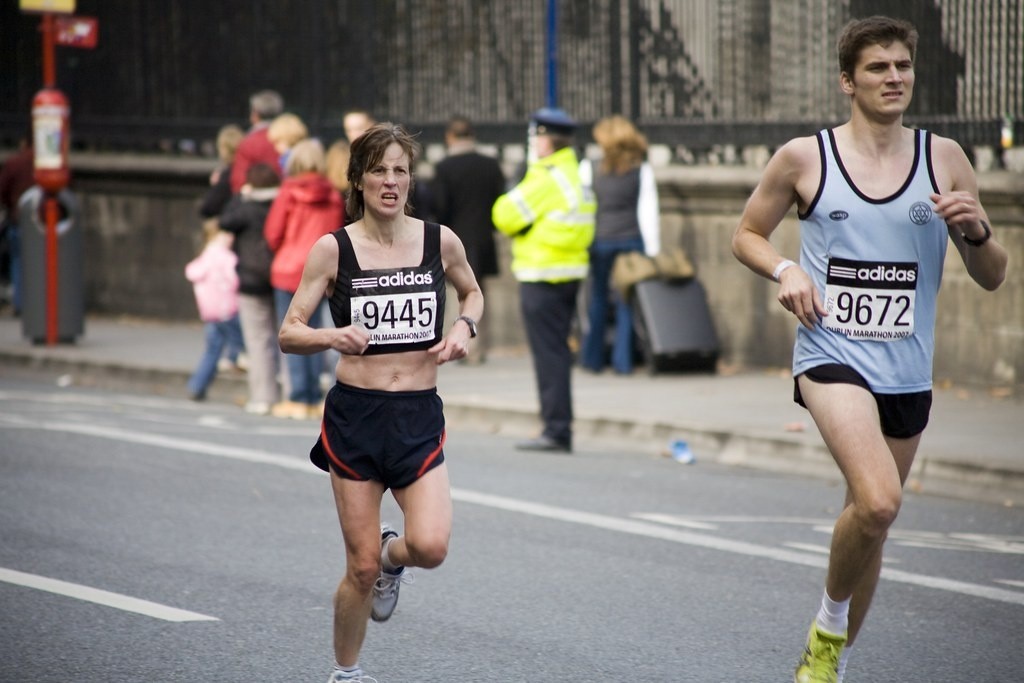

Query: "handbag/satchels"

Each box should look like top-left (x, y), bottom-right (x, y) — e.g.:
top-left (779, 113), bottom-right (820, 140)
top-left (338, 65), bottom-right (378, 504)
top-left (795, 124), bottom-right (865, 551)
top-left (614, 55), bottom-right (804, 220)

top-left (609, 247), bottom-right (693, 305)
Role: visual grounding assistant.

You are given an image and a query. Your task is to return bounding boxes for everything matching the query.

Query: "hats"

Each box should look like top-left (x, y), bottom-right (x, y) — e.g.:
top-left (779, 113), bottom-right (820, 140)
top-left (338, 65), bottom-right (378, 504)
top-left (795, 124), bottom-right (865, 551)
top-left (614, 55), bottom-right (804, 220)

top-left (527, 107), bottom-right (578, 139)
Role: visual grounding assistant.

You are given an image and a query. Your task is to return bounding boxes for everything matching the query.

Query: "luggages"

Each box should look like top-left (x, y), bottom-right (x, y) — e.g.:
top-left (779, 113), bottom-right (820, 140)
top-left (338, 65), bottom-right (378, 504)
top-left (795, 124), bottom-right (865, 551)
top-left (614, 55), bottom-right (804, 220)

top-left (630, 277), bottom-right (723, 377)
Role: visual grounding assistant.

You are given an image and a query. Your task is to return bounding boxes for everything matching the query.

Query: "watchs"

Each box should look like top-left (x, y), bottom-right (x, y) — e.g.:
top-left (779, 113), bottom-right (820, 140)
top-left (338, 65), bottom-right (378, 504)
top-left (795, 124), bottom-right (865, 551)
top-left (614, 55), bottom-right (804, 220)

top-left (454, 315), bottom-right (477, 338)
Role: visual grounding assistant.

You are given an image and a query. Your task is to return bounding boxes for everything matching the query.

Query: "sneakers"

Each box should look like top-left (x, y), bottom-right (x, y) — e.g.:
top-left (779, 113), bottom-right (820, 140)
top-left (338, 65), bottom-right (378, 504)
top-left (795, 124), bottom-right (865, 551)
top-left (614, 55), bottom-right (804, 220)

top-left (370, 523), bottom-right (414, 621)
top-left (794, 623), bottom-right (846, 683)
top-left (329, 667), bottom-right (376, 683)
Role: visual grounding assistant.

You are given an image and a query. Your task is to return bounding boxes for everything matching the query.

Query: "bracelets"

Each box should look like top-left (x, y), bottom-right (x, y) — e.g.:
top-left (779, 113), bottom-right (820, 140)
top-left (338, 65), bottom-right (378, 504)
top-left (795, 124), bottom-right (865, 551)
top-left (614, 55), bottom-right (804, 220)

top-left (961, 221), bottom-right (991, 246)
top-left (773, 260), bottom-right (795, 281)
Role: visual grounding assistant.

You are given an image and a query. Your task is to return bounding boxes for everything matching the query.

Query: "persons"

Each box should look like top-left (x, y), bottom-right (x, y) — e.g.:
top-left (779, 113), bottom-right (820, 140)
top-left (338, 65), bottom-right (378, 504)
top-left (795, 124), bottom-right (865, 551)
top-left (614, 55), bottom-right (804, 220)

top-left (432, 115), bottom-right (507, 367)
top-left (327, 113), bottom-right (378, 201)
top-left (185, 198), bottom-right (247, 400)
top-left (211, 126), bottom-right (245, 204)
top-left (279, 120), bottom-right (484, 683)
top-left (265, 136), bottom-right (346, 418)
top-left (220, 162), bottom-right (283, 415)
top-left (729, 16), bottom-right (1007, 683)
top-left (242, 113), bottom-right (309, 202)
top-left (407, 142), bottom-right (437, 222)
top-left (491, 108), bottom-right (597, 449)
top-left (230, 90), bottom-right (285, 204)
top-left (580, 114), bottom-right (661, 373)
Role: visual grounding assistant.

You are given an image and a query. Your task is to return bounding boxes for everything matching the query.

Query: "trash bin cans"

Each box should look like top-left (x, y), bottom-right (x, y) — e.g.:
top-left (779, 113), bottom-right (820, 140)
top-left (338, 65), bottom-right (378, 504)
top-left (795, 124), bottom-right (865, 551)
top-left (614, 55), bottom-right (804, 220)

top-left (16, 182), bottom-right (86, 346)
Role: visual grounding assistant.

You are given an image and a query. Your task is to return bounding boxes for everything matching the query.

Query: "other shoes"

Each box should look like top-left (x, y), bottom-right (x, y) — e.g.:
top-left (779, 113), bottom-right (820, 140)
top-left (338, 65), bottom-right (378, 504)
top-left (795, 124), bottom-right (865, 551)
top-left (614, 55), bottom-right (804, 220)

top-left (244, 401), bottom-right (272, 414)
top-left (515, 436), bottom-right (570, 453)
top-left (274, 401), bottom-right (324, 421)
